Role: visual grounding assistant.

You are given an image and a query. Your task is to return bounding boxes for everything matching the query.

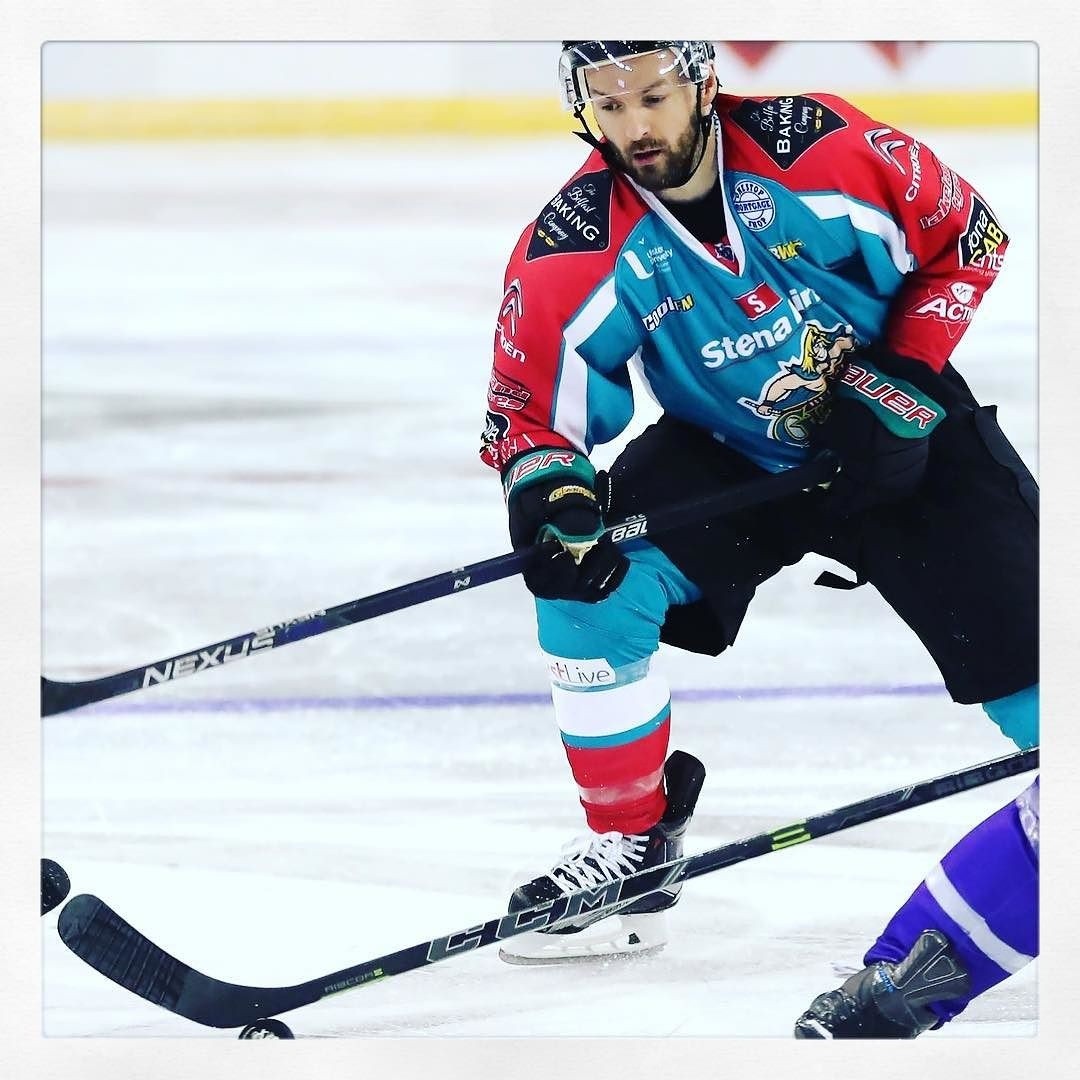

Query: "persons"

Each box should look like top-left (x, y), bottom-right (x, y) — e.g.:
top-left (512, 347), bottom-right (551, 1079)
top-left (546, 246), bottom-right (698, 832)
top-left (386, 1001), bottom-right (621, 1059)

top-left (478, 40), bottom-right (1040, 1038)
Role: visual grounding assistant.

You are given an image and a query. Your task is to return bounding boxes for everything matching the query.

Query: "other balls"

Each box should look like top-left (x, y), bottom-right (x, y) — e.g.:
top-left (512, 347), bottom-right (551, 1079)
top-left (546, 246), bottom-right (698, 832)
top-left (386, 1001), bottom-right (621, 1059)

top-left (239, 1019), bottom-right (294, 1038)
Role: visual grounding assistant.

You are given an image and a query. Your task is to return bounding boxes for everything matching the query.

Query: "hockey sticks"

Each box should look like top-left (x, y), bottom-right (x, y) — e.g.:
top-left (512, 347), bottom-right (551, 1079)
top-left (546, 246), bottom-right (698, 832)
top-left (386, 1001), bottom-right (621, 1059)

top-left (42, 399), bottom-right (1003, 723)
top-left (55, 745), bottom-right (1038, 1029)
top-left (40, 856), bottom-right (71, 916)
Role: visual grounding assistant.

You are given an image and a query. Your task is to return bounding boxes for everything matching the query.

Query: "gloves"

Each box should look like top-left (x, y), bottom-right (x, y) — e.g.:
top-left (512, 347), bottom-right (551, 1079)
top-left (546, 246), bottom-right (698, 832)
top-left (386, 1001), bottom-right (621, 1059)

top-left (795, 341), bottom-right (974, 531)
top-left (500, 445), bottom-right (632, 604)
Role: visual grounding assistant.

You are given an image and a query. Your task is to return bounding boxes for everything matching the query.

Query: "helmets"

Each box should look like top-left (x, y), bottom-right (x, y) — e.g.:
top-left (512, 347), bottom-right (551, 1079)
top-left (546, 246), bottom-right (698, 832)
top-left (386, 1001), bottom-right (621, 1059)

top-left (561, 40), bottom-right (715, 85)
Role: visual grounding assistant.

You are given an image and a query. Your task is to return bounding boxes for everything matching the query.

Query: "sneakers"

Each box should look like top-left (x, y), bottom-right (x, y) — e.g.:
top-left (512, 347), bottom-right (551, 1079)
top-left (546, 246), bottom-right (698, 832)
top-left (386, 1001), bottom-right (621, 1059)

top-left (498, 749), bottom-right (706, 965)
top-left (793, 928), bottom-right (973, 1041)
